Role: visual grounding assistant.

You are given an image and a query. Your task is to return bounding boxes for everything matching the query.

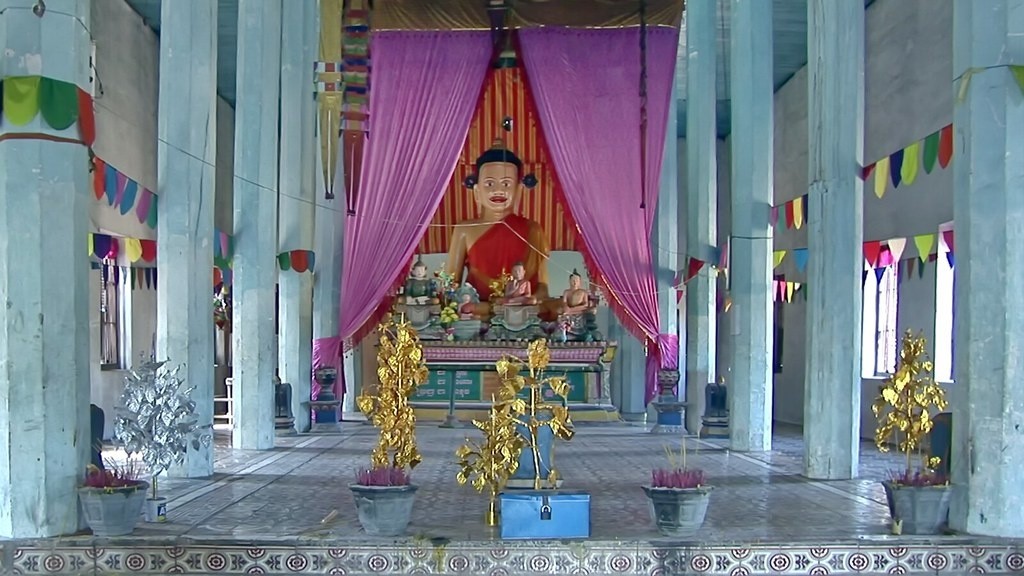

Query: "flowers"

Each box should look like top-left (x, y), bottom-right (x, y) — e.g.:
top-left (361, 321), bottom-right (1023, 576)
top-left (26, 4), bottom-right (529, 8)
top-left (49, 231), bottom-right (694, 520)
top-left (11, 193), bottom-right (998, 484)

top-left (652, 439), bottom-right (706, 488)
top-left (83, 457), bottom-right (142, 488)
top-left (885, 448), bottom-right (949, 487)
top-left (353, 464), bottom-right (411, 486)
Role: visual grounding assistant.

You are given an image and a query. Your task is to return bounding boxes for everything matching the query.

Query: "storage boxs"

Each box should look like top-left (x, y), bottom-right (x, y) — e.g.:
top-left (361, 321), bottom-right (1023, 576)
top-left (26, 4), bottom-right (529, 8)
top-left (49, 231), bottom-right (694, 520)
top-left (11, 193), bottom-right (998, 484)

top-left (499, 492), bottom-right (591, 540)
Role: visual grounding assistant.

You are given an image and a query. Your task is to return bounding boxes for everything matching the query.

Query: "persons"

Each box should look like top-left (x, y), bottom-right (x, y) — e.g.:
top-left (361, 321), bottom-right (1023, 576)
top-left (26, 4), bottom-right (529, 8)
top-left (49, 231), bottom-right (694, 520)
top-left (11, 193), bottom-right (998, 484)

top-left (405, 254), bottom-right (431, 305)
top-left (504, 260), bottom-right (537, 305)
top-left (457, 293), bottom-right (474, 320)
top-left (444, 138), bottom-right (564, 323)
top-left (563, 268), bottom-right (589, 314)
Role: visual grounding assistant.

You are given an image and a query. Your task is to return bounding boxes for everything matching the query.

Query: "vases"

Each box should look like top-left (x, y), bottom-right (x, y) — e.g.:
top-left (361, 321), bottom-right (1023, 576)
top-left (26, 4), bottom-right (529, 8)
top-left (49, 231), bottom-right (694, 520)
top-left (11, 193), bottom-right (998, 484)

top-left (881, 480), bottom-right (952, 534)
top-left (348, 482), bottom-right (419, 536)
top-left (75, 480), bottom-right (149, 536)
top-left (641, 485), bottom-right (716, 537)
